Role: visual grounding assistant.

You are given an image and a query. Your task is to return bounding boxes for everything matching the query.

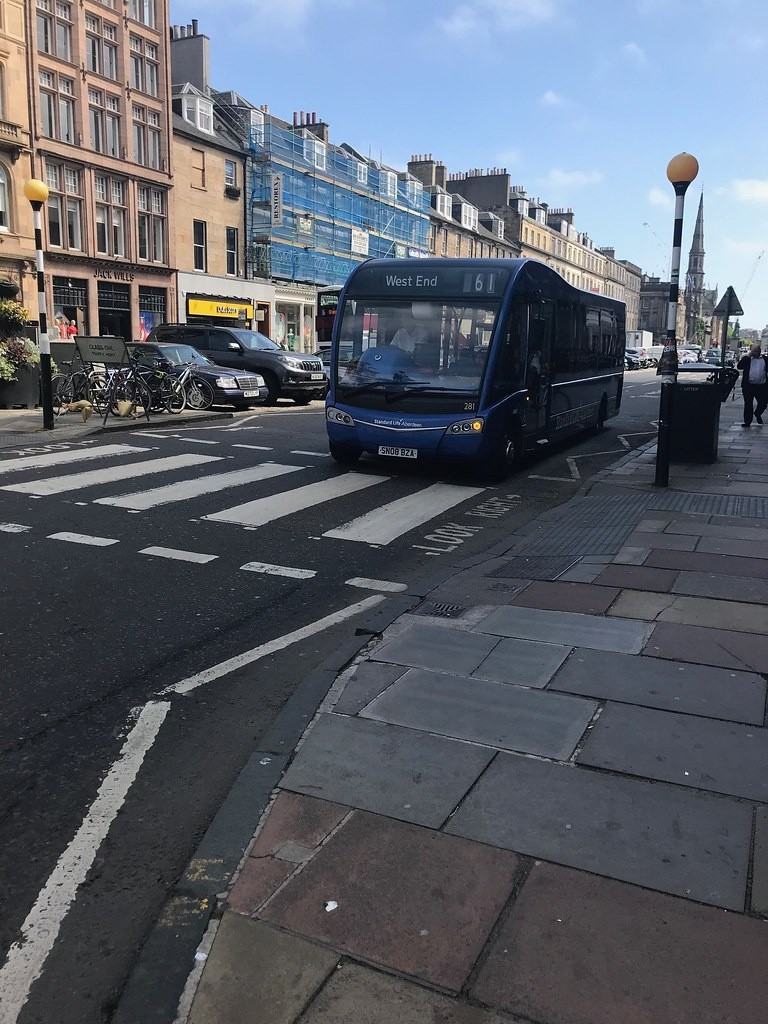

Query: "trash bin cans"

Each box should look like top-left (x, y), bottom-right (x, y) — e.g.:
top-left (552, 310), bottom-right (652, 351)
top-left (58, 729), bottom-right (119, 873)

top-left (670, 358), bottom-right (726, 464)
top-left (50, 338), bottom-right (105, 398)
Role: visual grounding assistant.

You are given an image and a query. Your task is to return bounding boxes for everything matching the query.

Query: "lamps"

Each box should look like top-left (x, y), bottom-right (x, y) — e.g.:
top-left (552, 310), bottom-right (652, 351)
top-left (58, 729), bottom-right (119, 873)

top-left (202, 293), bottom-right (252, 301)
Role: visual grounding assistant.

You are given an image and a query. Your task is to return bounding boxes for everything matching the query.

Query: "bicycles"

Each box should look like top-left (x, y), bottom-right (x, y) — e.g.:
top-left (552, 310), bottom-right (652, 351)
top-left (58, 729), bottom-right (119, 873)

top-left (52, 346), bottom-right (215, 417)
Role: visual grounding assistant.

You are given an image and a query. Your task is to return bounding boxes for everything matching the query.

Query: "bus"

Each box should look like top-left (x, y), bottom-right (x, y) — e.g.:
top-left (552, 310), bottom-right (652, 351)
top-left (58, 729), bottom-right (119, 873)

top-left (326, 256), bottom-right (625, 475)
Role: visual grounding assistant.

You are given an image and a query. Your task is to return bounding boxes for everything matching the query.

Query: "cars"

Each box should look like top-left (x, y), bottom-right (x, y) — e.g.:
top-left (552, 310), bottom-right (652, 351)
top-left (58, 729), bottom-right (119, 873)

top-left (126, 341), bottom-right (270, 409)
top-left (704, 350), bottom-right (737, 367)
top-left (312, 348), bottom-right (360, 399)
top-left (740, 347), bottom-right (768, 360)
top-left (676, 348), bottom-right (702, 367)
top-left (624, 348), bottom-right (649, 371)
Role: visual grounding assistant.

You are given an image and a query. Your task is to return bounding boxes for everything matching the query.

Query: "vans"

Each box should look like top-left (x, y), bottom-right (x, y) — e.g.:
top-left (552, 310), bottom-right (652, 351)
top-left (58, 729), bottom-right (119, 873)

top-left (642, 346), bottom-right (665, 368)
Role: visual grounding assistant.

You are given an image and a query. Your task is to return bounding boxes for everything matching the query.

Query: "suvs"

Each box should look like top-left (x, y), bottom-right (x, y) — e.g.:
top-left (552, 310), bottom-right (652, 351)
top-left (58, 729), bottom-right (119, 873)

top-left (147, 322), bottom-right (326, 406)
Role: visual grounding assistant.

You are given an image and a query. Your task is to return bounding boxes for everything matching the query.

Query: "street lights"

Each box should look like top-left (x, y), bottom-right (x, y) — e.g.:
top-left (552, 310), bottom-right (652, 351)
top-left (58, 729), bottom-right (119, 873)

top-left (23, 179), bottom-right (54, 429)
top-left (654, 152), bottom-right (699, 487)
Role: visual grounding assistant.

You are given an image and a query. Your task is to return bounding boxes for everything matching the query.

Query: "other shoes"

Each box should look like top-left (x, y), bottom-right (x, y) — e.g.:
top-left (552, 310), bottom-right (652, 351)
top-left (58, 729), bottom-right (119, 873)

top-left (755, 412), bottom-right (763, 424)
top-left (742, 424), bottom-right (750, 427)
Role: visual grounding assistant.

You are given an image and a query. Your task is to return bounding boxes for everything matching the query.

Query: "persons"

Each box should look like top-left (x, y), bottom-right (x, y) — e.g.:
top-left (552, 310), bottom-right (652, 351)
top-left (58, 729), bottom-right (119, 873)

top-left (390, 313), bottom-right (429, 354)
top-left (736, 343), bottom-right (768, 428)
top-left (55, 317), bottom-right (77, 340)
